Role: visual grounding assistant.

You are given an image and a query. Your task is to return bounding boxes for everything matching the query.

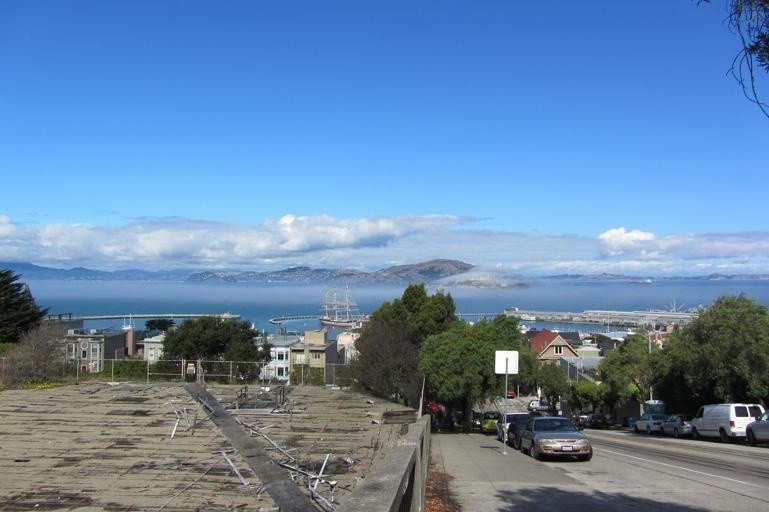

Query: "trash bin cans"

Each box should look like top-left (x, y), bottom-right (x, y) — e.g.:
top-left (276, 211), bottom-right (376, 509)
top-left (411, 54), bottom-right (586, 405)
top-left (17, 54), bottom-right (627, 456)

top-left (625, 415), bottom-right (633, 428)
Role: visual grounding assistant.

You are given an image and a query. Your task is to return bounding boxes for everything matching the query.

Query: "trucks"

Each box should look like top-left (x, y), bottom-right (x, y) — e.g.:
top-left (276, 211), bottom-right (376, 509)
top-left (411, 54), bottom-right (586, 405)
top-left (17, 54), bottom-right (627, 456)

top-left (690, 402), bottom-right (766, 441)
top-left (529, 399), bottom-right (551, 412)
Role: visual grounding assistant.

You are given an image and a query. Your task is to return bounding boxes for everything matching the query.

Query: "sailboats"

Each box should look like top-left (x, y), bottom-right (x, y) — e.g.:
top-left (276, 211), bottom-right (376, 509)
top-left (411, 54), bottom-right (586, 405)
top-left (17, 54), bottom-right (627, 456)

top-left (318, 278), bottom-right (360, 330)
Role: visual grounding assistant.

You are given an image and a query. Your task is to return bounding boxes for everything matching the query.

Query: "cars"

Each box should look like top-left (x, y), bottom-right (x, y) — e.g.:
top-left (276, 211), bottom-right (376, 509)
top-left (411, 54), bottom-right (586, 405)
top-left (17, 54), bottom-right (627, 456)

top-left (661, 413), bottom-right (694, 438)
top-left (506, 417), bottom-right (557, 449)
top-left (425, 398), bottom-right (503, 435)
top-left (520, 416), bottom-right (594, 463)
top-left (505, 390), bottom-right (515, 398)
top-left (632, 412), bottom-right (670, 434)
top-left (586, 413), bottom-right (608, 429)
top-left (745, 409), bottom-right (769, 447)
top-left (574, 415), bottom-right (587, 425)
top-left (496, 411), bottom-right (529, 443)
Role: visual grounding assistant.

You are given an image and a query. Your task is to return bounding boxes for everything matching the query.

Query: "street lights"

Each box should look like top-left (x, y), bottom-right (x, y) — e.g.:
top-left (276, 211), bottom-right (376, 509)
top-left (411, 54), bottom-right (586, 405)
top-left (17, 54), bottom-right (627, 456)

top-left (567, 362), bottom-right (575, 386)
top-left (645, 321), bottom-right (670, 399)
top-left (575, 356), bottom-right (585, 384)
top-left (258, 347), bottom-right (276, 376)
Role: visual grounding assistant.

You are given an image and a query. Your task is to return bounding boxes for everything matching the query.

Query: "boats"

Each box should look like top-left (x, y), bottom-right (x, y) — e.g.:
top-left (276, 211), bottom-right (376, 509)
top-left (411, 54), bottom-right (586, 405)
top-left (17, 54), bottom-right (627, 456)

top-left (520, 313), bottom-right (537, 321)
